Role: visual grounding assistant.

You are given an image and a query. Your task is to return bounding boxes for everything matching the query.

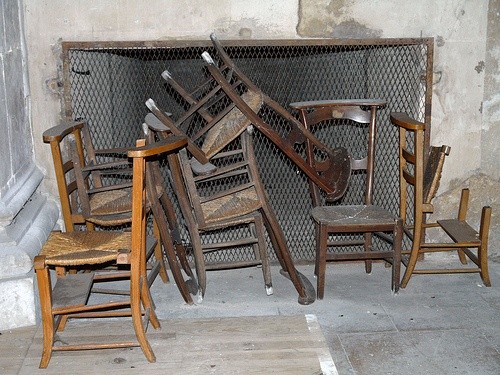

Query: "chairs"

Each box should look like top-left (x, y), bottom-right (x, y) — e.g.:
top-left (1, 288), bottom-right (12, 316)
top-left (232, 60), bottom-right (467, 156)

top-left (33, 32), bottom-right (492, 371)
